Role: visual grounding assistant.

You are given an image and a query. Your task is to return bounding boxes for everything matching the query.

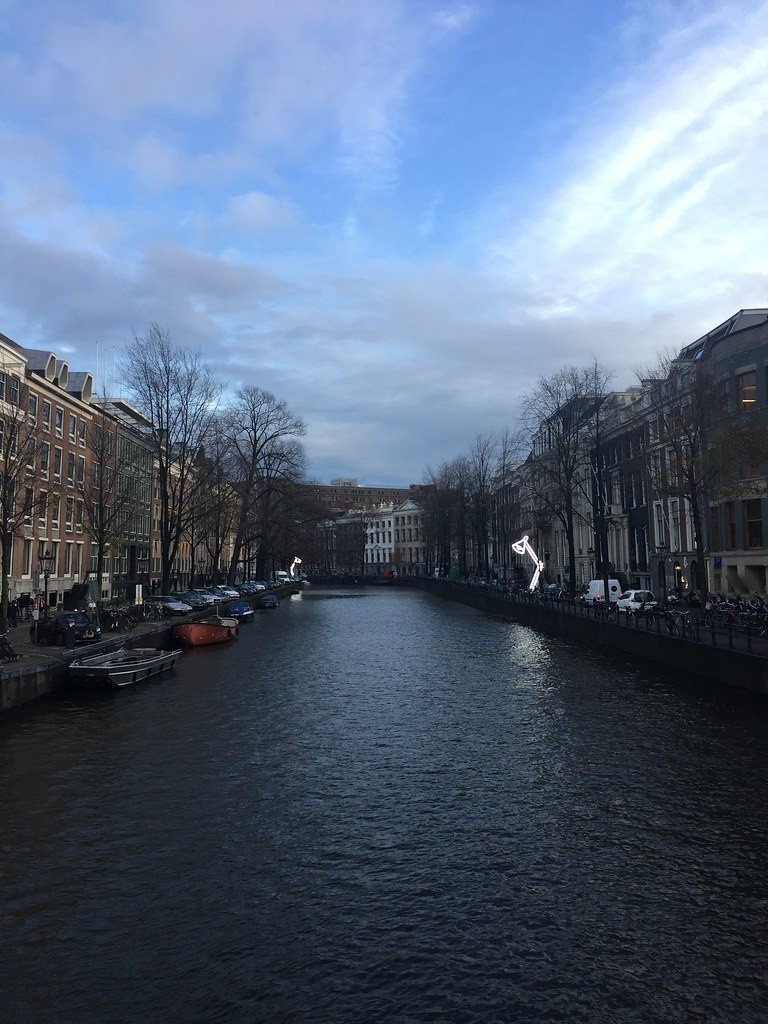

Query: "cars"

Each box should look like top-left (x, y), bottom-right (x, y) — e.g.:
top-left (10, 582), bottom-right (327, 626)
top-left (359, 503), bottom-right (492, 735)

top-left (236, 579), bottom-right (282, 597)
top-left (170, 591), bottom-right (209, 611)
top-left (217, 586), bottom-right (240, 600)
top-left (30, 611), bottom-right (102, 647)
top-left (185, 589), bottom-right (223, 606)
top-left (144, 596), bottom-right (193, 615)
top-left (615, 589), bottom-right (658, 615)
top-left (194, 587), bottom-right (231, 603)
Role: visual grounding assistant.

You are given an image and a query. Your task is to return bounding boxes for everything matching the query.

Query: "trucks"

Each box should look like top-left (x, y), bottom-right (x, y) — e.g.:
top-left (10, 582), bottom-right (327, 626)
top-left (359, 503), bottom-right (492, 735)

top-left (583, 580), bottom-right (623, 605)
top-left (271, 571), bottom-right (290, 583)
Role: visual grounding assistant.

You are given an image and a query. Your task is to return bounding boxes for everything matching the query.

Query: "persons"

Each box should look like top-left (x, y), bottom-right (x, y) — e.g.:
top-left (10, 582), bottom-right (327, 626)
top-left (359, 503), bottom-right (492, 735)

top-left (8, 592), bottom-right (44, 629)
top-left (435, 562), bottom-right (449, 579)
top-left (688, 586), bottom-right (697, 602)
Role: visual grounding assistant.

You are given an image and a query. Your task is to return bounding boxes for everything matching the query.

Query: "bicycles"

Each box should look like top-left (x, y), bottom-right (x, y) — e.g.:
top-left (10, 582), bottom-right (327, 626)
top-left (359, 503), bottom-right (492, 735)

top-left (639, 587), bottom-right (768, 641)
top-left (0, 632), bottom-right (15, 664)
top-left (100, 600), bottom-right (172, 634)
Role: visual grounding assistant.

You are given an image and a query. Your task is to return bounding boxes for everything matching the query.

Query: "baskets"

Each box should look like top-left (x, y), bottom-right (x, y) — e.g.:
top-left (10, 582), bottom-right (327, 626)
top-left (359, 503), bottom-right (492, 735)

top-left (706, 602), bottom-right (717, 610)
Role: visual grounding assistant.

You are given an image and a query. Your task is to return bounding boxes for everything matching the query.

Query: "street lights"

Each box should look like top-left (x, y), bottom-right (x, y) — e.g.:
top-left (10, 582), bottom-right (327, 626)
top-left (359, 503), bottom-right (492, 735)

top-left (38, 549), bottom-right (56, 644)
top-left (586, 547), bottom-right (596, 580)
top-left (250, 561), bottom-right (255, 575)
top-left (198, 558), bottom-right (205, 586)
top-left (544, 550), bottom-right (551, 574)
top-left (656, 542), bottom-right (670, 625)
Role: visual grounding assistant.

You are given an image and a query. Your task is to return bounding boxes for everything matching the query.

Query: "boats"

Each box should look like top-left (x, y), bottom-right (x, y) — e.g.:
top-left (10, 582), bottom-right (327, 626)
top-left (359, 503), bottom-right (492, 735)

top-left (170, 614), bottom-right (239, 647)
top-left (67, 647), bottom-right (182, 694)
top-left (259, 595), bottom-right (279, 608)
top-left (225, 602), bottom-right (255, 624)
